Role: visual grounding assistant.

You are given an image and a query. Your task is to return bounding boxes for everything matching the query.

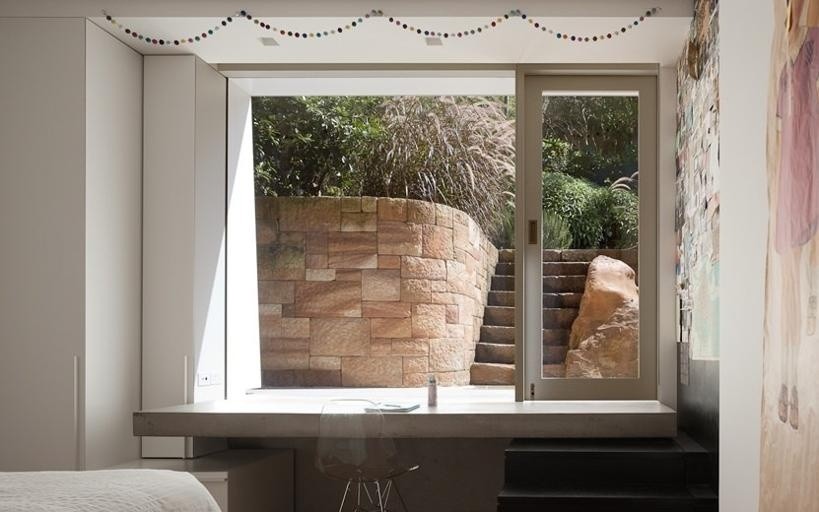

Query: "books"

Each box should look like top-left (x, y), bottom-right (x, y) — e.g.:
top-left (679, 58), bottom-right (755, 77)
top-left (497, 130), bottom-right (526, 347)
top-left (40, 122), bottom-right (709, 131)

top-left (364, 401), bottom-right (421, 413)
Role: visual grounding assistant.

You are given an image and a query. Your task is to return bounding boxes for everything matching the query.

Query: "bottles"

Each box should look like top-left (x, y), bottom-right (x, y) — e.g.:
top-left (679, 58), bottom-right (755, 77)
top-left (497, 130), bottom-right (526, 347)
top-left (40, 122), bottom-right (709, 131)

top-left (427, 376), bottom-right (437, 407)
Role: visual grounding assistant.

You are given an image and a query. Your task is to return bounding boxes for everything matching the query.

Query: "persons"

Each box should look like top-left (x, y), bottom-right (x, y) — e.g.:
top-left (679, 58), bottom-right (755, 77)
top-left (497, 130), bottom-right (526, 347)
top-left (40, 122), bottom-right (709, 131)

top-left (764, 0), bottom-right (819, 428)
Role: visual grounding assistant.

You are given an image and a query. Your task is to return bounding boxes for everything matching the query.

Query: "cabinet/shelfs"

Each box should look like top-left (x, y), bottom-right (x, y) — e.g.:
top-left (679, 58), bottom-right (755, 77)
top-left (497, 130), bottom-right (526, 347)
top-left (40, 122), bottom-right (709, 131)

top-left (103, 449), bottom-right (295, 511)
top-left (2, 17), bottom-right (143, 469)
